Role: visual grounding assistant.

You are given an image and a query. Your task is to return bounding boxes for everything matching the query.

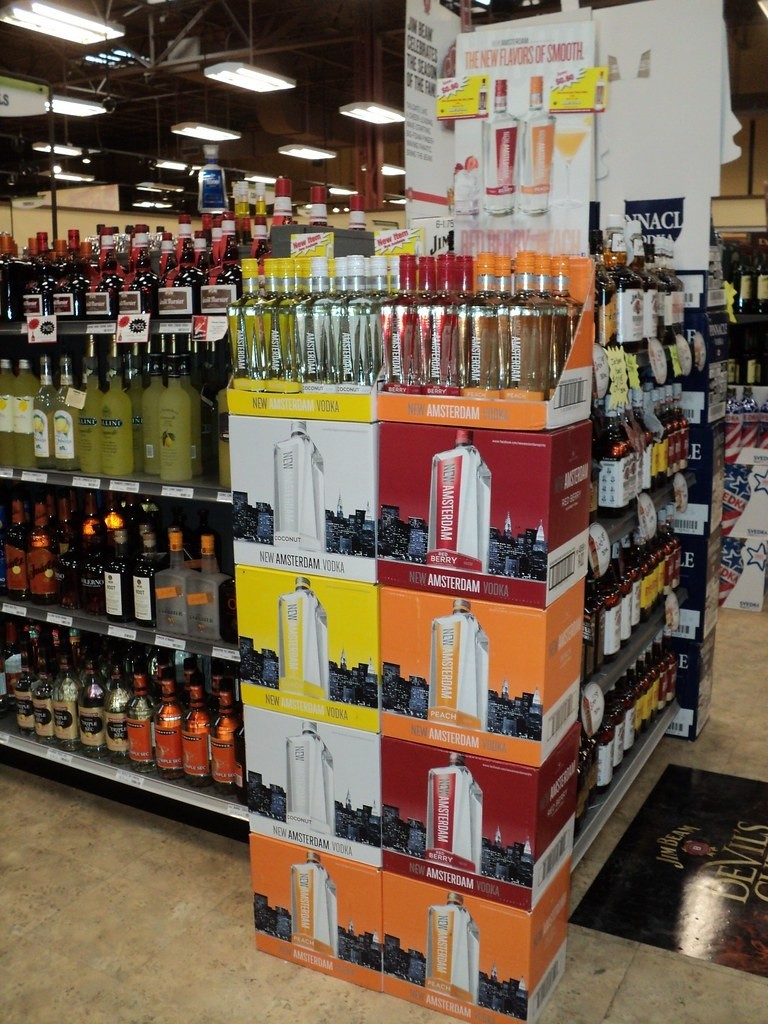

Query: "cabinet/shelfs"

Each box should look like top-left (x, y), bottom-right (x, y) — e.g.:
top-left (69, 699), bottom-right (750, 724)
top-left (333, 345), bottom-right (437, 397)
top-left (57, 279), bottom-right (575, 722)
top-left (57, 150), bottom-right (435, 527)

top-left (570, 251), bottom-right (685, 875)
top-left (0, 223), bottom-right (375, 830)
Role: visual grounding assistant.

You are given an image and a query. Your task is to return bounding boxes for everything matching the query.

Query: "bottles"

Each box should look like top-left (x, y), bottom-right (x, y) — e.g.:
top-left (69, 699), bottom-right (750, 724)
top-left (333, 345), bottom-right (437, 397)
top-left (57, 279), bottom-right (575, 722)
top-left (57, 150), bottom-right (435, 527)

top-left (0, 177), bottom-right (365, 322)
top-left (272, 422), bottom-right (328, 553)
top-left (426, 430), bottom-right (491, 569)
top-left (428, 752), bottom-right (483, 875)
top-left (286, 719), bottom-right (336, 836)
top-left (229, 250), bottom-right (574, 400)
top-left (722, 238), bottom-right (768, 384)
top-left (427, 600), bottom-right (488, 734)
top-left (478, 81), bottom-right (523, 217)
top-left (0, 333), bottom-right (231, 492)
top-left (275, 579), bottom-right (328, 698)
top-left (426, 892), bottom-right (480, 1006)
top-left (0, 493), bottom-right (234, 642)
top-left (291, 852), bottom-right (338, 961)
top-left (525, 76), bottom-right (555, 212)
top-left (0, 618), bottom-right (246, 805)
top-left (577, 243), bottom-right (688, 835)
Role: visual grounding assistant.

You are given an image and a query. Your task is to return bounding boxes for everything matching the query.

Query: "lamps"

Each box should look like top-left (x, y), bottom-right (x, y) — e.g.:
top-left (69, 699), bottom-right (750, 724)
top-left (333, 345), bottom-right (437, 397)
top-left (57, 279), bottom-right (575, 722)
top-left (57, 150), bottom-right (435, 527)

top-left (0, 0), bottom-right (408, 213)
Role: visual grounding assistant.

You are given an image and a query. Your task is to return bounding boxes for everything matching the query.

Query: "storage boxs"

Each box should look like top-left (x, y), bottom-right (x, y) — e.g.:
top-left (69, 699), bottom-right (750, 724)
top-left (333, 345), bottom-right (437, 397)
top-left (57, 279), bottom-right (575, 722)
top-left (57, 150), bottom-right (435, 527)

top-left (229, 414), bottom-right (598, 1024)
top-left (716, 384), bottom-right (768, 613)
